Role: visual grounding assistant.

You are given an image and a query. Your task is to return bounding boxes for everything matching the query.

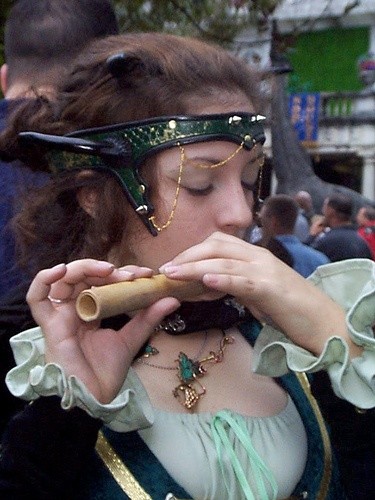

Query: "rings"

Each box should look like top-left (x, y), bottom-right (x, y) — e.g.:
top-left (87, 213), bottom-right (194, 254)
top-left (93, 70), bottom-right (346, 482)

top-left (48, 295), bottom-right (72, 304)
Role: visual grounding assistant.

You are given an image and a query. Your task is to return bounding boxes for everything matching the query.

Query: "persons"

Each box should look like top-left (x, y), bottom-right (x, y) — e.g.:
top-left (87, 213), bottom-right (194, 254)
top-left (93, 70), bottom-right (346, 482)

top-left (294, 191), bottom-right (324, 225)
top-left (303, 193), bottom-right (373, 262)
top-left (1, 31), bottom-right (375, 500)
top-left (250, 197), bottom-right (264, 243)
top-left (253, 194), bottom-right (331, 277)
top-left (1, 1), bottom-right (121, 336)
top-left (356, 204), bottom-right (375, 260)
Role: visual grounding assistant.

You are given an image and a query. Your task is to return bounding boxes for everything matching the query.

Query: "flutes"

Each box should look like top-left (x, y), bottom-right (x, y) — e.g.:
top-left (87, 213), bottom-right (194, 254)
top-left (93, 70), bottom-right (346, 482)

top-left (76, 274), bottom-right (213, 321)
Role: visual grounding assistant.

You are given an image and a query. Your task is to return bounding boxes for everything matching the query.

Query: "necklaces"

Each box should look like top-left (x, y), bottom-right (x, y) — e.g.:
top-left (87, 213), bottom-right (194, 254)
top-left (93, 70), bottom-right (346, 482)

top-left (135, 327), bottom-right (236, 410)
